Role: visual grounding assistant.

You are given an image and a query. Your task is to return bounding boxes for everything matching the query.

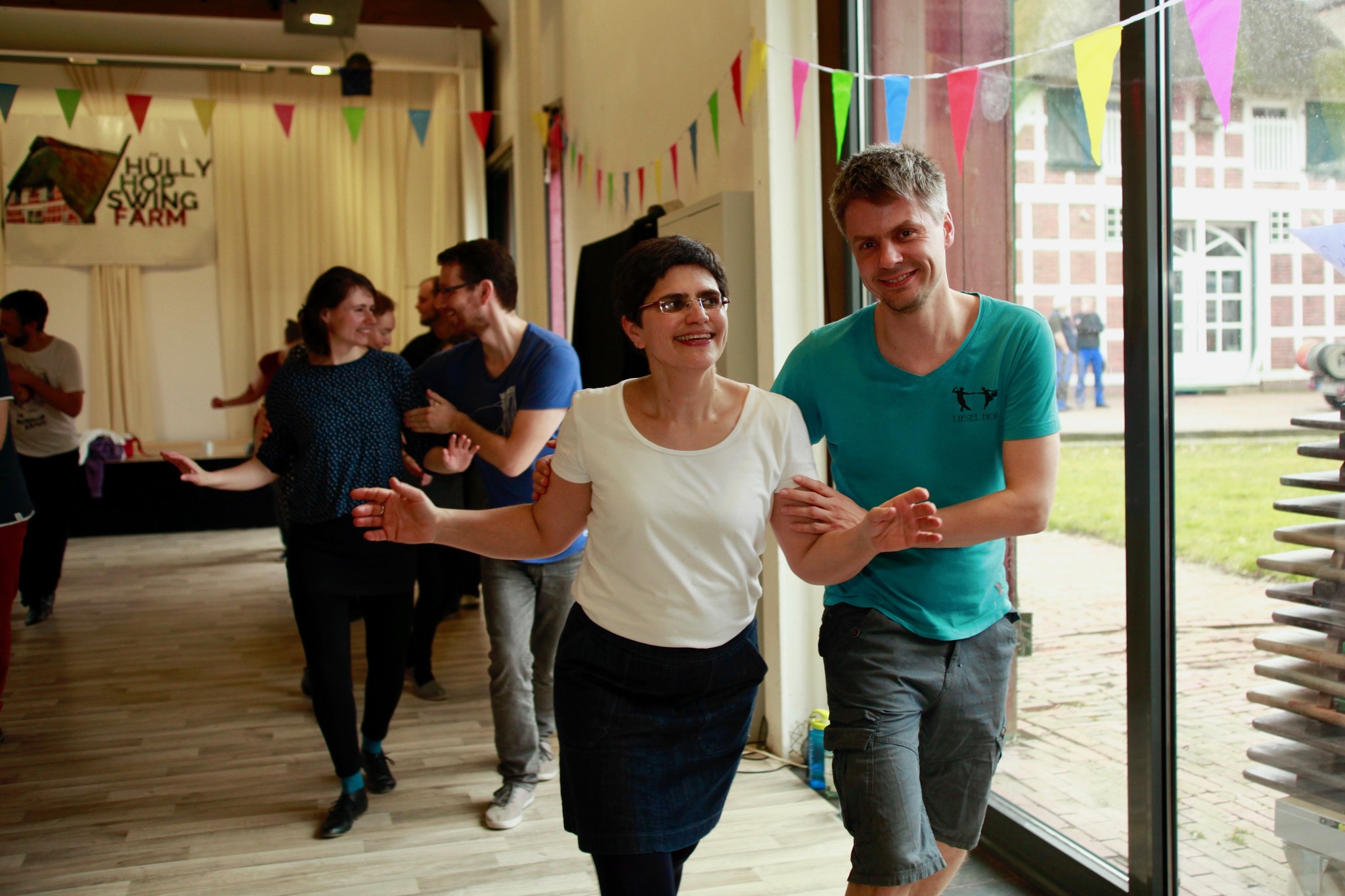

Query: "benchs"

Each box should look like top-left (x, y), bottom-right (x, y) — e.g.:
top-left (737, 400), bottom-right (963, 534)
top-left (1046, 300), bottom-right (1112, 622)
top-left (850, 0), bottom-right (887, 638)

top-left (67, 441), bottom-right (276, 537)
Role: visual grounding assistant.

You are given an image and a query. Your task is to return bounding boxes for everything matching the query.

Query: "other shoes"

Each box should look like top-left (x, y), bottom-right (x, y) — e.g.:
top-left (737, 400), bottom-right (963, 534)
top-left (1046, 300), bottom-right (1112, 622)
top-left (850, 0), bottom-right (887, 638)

top-left (412, 677), bottom-right (447, 700)
top-left (25, 593), bottom-right (54, 624)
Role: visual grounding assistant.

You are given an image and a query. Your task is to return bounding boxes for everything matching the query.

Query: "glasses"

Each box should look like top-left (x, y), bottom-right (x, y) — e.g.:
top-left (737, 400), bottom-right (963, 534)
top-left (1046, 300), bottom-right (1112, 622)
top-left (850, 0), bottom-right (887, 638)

top-left (638, 295), bottom-right (730, 314)
top-left (438, 279), bottom-right (486, 295)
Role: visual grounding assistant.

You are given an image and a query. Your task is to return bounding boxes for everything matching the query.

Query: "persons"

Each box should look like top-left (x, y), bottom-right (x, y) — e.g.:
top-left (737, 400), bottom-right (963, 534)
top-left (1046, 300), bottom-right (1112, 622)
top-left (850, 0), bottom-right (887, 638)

top-left (212, 319), bottom-right (304, 408)
top-left (398, 276), bottom-right (466, 365)
top-left (0, 289), bottom-right (85, 628)
top-left (403, 238), bottom-right (584, 829)
top-left (349, 234), bottom-right (943, 896)
top-left (1049, 295), bottom-right (1078, 412)
top-left (768, 144), bottom-right (1062, 896)
top-left (158, 265), bottom-right (481, 839)
top-left (1073, 295), bottom-right (1108, 409)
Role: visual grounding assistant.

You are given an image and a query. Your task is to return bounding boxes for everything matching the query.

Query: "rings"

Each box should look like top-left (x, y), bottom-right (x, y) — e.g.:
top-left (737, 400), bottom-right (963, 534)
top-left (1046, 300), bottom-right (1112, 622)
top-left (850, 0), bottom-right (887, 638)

top-left (380, 505), bottom-right (384, 515)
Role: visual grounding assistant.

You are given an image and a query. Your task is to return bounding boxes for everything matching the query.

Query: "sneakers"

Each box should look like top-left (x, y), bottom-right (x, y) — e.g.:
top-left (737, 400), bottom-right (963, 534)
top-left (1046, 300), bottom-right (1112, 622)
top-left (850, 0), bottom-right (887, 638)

top-left (536, 740), bottom-right (555, 781)
top-left (361, 745), bottom-right (395, 792)
top-left (485, 782), bottom-right (536, 830)
top-left (320, 787), bottom-right (367, 838)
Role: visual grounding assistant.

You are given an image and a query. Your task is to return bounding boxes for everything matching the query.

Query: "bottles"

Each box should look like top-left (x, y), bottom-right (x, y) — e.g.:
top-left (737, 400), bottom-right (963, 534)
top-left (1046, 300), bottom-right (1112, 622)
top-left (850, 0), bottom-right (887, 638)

top-left (807, 720), bottom-right (840, 801)
